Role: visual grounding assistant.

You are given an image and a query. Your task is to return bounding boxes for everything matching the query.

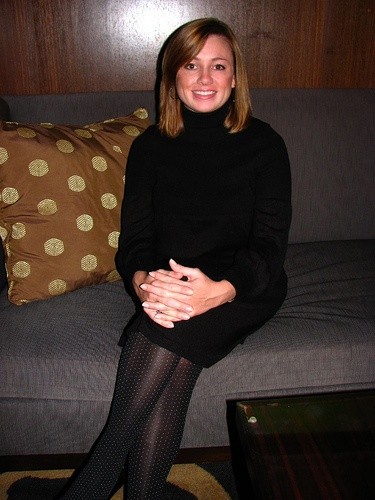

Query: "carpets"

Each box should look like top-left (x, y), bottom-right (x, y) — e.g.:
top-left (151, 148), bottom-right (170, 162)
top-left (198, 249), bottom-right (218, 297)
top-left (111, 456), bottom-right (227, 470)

top-left (0, 463), bottom-right (232, 500)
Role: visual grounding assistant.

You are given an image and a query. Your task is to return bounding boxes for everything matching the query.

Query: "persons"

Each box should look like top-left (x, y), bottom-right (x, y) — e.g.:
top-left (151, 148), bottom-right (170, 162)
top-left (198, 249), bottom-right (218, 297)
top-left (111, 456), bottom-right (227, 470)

top-left (61, 19), bottom-right (293, 500)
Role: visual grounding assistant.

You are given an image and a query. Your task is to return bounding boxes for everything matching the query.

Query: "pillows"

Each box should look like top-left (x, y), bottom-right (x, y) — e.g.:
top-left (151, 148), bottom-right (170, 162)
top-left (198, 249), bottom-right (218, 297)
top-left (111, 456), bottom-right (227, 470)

top-left (0, 109), bottom-right (152, 306)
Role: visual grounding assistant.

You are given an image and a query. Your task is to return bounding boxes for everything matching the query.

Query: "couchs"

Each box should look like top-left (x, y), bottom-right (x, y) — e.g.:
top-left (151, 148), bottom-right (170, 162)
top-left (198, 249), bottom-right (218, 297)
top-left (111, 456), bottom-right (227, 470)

top-left (1, 87), bottom-right (375, 455)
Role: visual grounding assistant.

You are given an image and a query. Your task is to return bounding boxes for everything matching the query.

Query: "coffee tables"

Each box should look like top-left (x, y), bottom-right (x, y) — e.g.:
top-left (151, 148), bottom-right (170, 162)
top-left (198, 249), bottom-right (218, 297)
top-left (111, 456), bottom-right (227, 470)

top-left (237, 391), bottom-right (375, 500)
